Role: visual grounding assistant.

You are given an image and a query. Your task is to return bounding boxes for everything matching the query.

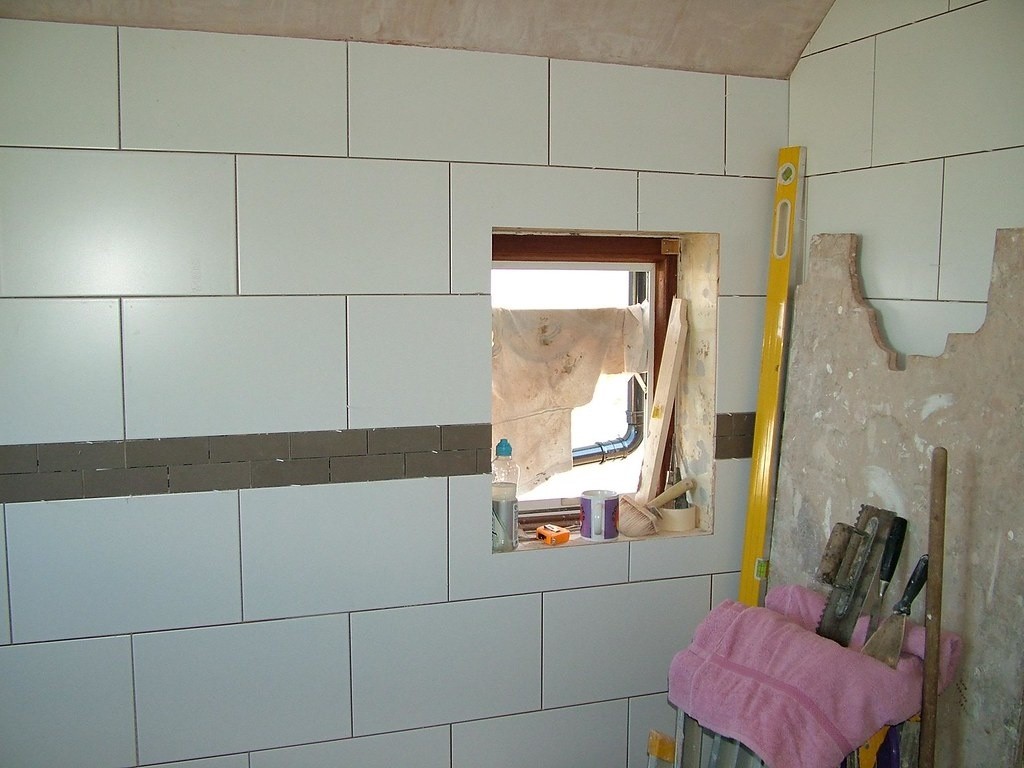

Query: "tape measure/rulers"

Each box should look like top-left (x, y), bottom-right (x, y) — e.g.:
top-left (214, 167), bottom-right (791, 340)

top-left (536, 524), bottom-right (571, 546)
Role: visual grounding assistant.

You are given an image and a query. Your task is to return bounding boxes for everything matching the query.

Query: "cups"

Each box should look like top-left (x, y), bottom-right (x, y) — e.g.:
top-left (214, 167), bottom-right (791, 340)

top-left (579, 489), bottom-right (619, 541)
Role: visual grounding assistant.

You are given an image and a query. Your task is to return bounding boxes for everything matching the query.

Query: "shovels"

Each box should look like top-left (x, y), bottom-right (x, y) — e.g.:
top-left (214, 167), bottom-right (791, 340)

top-left (858, 554), bottom-right (931, 670)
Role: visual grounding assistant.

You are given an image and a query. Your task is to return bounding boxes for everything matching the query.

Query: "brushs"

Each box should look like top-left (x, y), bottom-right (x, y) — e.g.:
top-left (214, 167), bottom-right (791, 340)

top-left (617, 477), bottom-right (695, 537)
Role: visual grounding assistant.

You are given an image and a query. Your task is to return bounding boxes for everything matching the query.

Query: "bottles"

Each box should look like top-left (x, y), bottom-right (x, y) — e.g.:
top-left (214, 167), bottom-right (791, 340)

top-left (675, 467), bottom-right (688, 509)
top-left (492, 439), bottom-right (519, 551)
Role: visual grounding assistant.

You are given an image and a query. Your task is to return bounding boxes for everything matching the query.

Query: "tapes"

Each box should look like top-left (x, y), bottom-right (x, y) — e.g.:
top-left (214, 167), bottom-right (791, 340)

top-left (656, 503), bottom-right (696, 531)
